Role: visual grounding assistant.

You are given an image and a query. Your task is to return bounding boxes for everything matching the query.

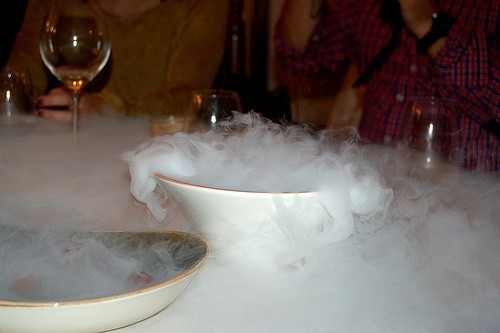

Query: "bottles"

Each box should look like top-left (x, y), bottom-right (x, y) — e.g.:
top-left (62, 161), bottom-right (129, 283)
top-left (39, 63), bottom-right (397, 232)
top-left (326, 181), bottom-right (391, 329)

top-left (212, 16), bottom-right (248, 115)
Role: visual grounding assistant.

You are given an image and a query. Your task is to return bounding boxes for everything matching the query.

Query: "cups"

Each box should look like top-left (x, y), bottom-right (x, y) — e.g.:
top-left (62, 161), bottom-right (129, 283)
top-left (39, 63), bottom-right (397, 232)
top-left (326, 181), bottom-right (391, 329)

top-left (149, 106), bottom-right (199, 136)
top-left (0, 65), bottom-right (35, 128)
top-left (185, 89), bottom-right (242, 135)
top-left (407, 95), bottom-right (456, 179)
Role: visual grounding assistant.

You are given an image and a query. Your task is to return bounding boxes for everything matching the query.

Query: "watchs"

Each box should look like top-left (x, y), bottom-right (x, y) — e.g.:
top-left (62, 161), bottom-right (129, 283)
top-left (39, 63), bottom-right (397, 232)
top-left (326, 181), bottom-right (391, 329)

top-left (418, 13), bottom-right (456, 52)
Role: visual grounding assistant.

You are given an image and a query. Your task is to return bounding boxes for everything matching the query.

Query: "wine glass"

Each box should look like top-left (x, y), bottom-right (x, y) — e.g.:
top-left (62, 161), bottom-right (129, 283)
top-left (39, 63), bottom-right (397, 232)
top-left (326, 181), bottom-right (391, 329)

top-left (39, 4), bottom-right (113, 140)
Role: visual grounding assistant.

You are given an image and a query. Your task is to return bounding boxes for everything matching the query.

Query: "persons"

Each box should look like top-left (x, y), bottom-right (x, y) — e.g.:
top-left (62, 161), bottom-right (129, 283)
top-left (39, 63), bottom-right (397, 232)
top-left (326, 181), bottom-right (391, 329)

top-left (0, 0), bottom-right (230, 136)
top-left (272, 0), bottom-right (500, 174)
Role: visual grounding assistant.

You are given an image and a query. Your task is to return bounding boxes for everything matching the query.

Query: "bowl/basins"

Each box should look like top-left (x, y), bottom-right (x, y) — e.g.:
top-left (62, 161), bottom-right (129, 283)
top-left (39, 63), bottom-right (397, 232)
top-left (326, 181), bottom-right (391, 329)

top-left (135, 139), bottom-right (344, 253)
top-left (0, 224), bottom-right (210, 333)
